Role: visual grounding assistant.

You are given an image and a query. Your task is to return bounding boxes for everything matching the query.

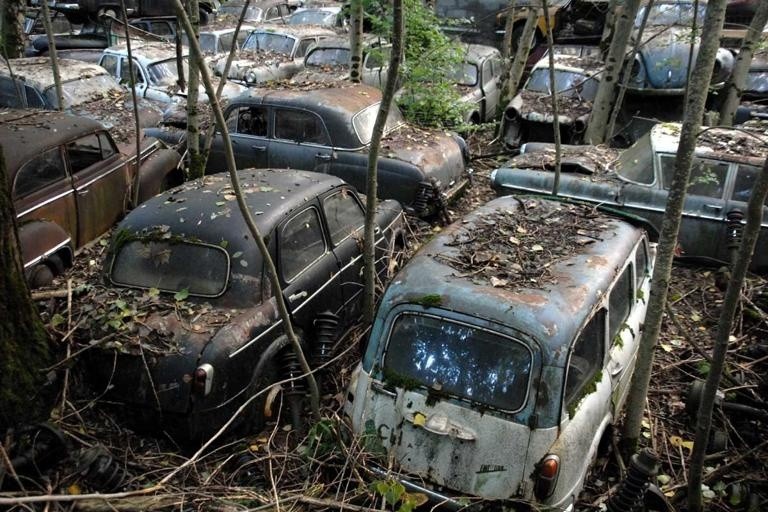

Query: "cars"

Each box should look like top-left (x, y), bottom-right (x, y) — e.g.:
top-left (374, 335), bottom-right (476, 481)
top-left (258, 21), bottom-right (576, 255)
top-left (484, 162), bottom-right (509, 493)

top-left (95, 42), bottom-right (249, 114)
top-left (344, 188), bottom-right (658, 511)
top-left (391, 42), bottom-right (511, 140)
top-left (0, 55), bottom-right (164, 160)
top-left (213, 25), bottom-right (338, 87)
top-left (601, 0), bottom-right (736, 104)
top-left (707, 22), bottom-right (768, 127)
top-left (142, 78), bottom-right (475, 217)
top-left (289, 32), bottom-right (409, 93)
top-left (427, 0), bottom-right (609, 61)
top-left (22, 0), bottom-right (350, 41)
top-left (0, 106), bottom-right (187, 297)
top-left (486, 122), bottom-right (768, 276)
top-left (496, 44), bottom-right (632, 152)
top-left (73, 167), bottom-right (406, 444)
